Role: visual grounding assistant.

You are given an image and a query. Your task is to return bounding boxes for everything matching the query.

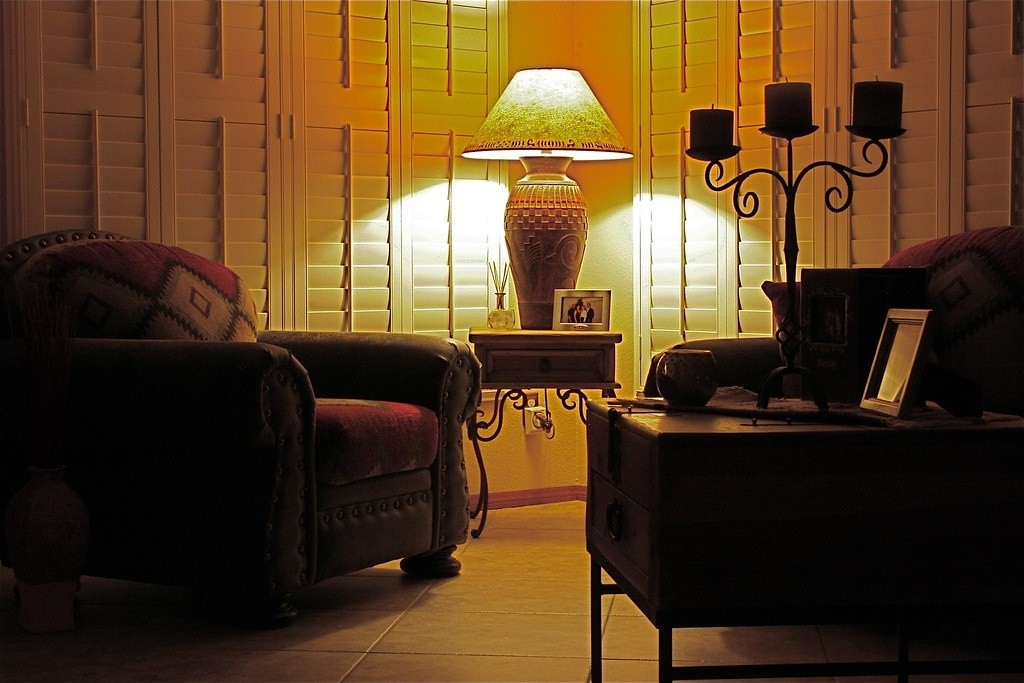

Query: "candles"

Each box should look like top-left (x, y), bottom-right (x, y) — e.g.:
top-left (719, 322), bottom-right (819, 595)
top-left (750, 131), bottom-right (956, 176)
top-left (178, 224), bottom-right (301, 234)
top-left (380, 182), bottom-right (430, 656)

top-left (766, 77), bottom-right (812, 129)
top-left (687, 103), bottom-right (735, 148)
top-left (853, 75), bottom-right (904, 129)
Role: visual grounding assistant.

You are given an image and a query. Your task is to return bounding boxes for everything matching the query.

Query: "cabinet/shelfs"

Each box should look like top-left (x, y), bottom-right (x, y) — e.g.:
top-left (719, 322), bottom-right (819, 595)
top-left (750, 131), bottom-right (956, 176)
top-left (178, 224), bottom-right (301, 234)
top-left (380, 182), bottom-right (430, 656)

top-left (586, 400), bottom-right (1023, 683)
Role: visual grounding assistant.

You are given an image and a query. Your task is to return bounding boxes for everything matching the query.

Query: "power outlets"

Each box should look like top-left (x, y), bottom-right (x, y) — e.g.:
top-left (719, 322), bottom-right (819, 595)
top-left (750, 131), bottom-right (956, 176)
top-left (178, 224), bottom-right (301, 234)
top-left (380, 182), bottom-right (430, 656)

top-left (523, 392), bottom-right (539, 426)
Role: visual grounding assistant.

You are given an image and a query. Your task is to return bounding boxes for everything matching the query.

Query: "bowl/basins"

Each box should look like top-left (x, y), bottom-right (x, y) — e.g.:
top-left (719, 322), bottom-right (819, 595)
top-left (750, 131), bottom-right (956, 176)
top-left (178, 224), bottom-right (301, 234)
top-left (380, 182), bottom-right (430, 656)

top-left (657, 351), bottom-right (718, 407)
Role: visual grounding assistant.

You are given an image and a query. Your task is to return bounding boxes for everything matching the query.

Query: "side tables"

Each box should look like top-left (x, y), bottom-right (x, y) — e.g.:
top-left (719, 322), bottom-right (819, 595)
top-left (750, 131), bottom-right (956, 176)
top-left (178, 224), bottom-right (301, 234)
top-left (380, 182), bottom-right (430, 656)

top-left (468, 326), bottom-right (623, 538)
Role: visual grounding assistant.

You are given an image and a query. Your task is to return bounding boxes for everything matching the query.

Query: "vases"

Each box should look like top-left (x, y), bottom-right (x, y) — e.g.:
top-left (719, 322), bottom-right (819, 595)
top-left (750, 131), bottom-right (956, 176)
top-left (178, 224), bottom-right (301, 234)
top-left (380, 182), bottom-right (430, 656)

top-left (487, 292), bottom-right (515, 330)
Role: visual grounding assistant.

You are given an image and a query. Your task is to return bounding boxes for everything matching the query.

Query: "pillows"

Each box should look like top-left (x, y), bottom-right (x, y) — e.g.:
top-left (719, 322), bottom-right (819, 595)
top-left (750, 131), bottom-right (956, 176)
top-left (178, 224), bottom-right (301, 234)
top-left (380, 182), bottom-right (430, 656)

top-left (761, 281), bottom-right (802, 398)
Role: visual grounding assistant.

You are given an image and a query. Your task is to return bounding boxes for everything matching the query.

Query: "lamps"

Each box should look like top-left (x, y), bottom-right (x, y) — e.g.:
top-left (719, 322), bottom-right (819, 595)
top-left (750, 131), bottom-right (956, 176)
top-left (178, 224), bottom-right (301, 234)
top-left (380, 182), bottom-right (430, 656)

top-left (459, 67), bottom-right (634, 330)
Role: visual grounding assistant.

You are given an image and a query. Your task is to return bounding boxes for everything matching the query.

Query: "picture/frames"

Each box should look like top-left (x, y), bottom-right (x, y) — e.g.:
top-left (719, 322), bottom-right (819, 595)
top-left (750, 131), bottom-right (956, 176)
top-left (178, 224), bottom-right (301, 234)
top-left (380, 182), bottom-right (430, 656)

top-left (552, 290), bottom-right (612, 331)
top-left (859, 308), bottom-right (932, 419)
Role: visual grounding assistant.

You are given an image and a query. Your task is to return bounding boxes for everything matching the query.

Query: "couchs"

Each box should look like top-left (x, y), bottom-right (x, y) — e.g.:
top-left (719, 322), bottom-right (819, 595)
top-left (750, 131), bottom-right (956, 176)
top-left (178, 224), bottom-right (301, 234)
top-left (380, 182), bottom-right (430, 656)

top-left (0, 229), bottom-right (482, 647)
top-left (651, 228), bottom-right (1022, 410)
